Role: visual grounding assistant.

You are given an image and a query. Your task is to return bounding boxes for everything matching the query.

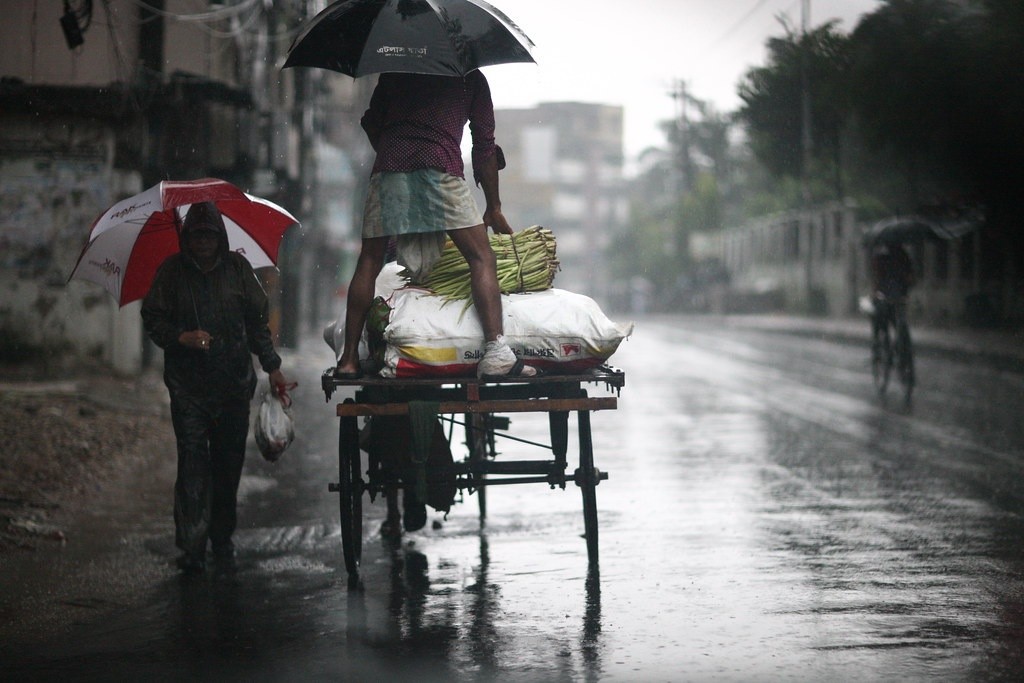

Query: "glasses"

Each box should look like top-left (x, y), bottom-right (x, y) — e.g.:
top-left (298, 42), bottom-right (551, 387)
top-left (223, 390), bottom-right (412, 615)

top-left (190, 230), bottom-right (219, 240)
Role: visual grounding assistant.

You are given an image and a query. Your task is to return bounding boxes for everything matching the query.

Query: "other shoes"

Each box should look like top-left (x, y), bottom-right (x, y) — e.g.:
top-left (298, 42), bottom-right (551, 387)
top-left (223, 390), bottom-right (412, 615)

top-left (213, 552), bottom-right (238, 577)
top-left (175, 552), bottom-right (205, 576)
top-left (379, 512), bottom-right (402, 536)
top-left (401, 485), bottom-right (427, 533)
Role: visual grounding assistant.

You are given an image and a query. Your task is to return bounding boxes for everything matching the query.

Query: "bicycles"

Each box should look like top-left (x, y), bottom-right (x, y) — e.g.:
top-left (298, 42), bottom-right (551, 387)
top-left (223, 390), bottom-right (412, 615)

top-left (871, 282), bottom-right (914, 403)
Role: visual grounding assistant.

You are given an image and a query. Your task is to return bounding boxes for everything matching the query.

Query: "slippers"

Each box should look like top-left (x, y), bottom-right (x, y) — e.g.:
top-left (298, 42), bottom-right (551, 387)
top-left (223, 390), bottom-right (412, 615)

top-left (481, 359), bottom-right (550, 381)
top-left (332, 359), bottom-right (364, 379)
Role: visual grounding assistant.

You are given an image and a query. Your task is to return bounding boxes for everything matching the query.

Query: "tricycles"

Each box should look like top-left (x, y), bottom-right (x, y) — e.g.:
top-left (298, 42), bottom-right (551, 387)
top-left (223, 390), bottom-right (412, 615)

top-left (318, 360), bottom-right (626, 578)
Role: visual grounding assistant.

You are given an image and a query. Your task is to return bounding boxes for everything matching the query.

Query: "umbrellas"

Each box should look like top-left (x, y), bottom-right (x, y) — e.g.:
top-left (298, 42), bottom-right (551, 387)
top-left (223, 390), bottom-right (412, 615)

top-left (64, 173), bottom-right (300, 332)
top-left (279, 0), bottom-right (540, 84)
top-left (860, 207), bottom-right (958, 248)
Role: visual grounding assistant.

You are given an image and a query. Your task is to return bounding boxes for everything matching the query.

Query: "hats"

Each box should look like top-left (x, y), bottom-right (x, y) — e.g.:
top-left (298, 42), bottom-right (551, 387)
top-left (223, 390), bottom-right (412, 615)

top-left (187, 222), bottom-right (220, 234)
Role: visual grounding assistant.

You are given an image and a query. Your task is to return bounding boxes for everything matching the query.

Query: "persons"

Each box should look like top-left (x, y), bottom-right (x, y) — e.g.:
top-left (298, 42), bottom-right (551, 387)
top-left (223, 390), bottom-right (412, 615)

top-left (139, 202), bottom-right (287, 575)
top-left (865, 242), bottom-right (922, 379)
top-left (333, 70), bottom-right (514, 376)
top-left (380, 412), bottom-right (428, 546)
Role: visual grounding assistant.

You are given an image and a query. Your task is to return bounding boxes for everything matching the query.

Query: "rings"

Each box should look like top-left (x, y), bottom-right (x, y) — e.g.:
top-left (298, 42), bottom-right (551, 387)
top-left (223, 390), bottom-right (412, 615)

top-left (201, 340), bottom-right (205, 345)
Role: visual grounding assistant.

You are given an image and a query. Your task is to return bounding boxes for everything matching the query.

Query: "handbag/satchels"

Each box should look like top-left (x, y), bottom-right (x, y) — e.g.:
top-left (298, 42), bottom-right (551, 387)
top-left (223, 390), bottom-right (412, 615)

top-left (254, 381), bottom-right (298, 463)
